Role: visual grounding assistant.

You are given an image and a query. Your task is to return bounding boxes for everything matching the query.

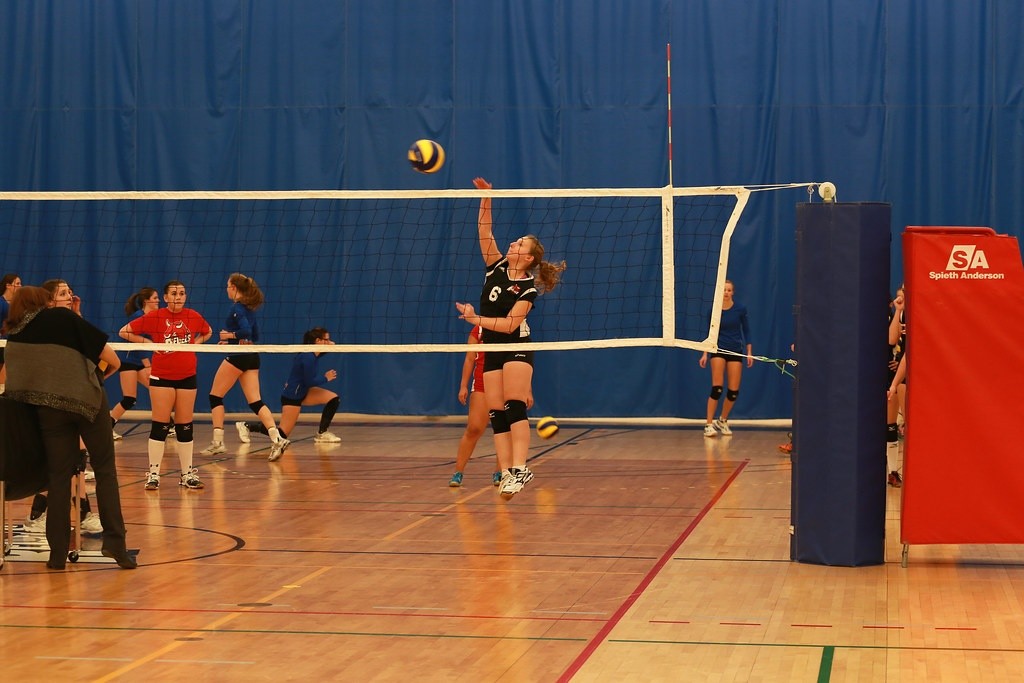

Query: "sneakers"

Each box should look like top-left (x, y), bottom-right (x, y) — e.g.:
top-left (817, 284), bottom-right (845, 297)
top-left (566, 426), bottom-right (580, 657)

top-left (236, 421), bottom-right (251, 443)
top-left (888, 471), bottom-right (902, 488)
top-left (80, 512), bottom-right (104, 535)
top-left (498, 470), bottom-right (515, 501)
top-left (268, 437), bottom-right (291, 461)
top-left (703, 424), bottom-right (717, 436)
top-left (493, 472), bottom-right (502, 485)
top-left (449, 472), bottom-right (463, 487)
top-left (23, 512), bottom-right (47, 532)
top-left (314, 430), bottom-right (341, 443)
top-left (502, 466), bottom-right (533, 494)
top-left (200, 441), bottom-right (226, 455)
top-left (178, 468), bottom-right (205, 488)
top-left (145, 472), bottom-right (160, 490)
top-left (715, 418), bottom-right (732, 434)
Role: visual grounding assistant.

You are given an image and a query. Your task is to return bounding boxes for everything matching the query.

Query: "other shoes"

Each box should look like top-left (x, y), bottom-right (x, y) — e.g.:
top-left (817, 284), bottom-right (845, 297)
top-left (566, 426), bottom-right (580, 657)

top-left (167, 426), bottom-right (175, 436)
top-left (101, 548), bottom-right (136, 569)
top-left (779, 441), bottom-right (792, 454)
top-left (85, 469), bottom-right (94, 480)
top-left (113, 430), bottom-right (122, 439)
top-left (46, 560), bottom-right (65, 570)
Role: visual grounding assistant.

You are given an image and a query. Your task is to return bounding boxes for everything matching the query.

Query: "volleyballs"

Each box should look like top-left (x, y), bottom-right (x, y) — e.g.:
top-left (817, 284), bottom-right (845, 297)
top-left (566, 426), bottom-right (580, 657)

top-left (407, 139), bottom-right (445, 175)
top-left (535, 416), bottom-right (560, 442)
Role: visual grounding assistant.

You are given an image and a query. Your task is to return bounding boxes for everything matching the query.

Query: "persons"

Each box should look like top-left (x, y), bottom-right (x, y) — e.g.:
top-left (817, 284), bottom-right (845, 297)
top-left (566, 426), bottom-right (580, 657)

top-left (235, 327), bottom-right (344, 443)
top-left (199, 273), bottom-right (291, 461)
top-left (448, 325), bottom-right (533, 488)
top-left (109, 287), bottom-right (177, 440)
top-left (120, 281), bottom-right (212, 488)
top-left (0, 273), bottom-right (140, 569)
top-left (777, 282), bottom-right (905, 487)
top-left (699, 280), bottom-right (753, 436)
top-left (456, 176), bottom-right (566, 503)
